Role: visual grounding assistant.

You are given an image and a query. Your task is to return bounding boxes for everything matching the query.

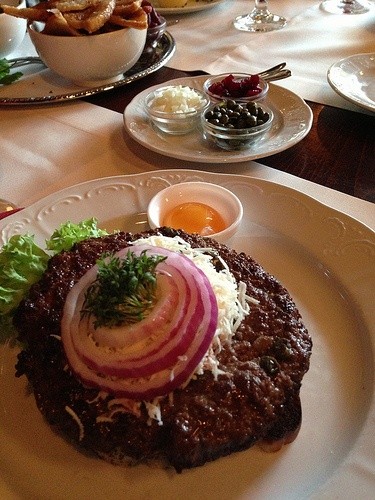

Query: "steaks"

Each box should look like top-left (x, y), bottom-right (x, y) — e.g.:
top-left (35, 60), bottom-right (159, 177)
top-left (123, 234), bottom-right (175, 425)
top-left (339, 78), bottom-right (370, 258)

top-left (14, 226), bottom-right (313, 472)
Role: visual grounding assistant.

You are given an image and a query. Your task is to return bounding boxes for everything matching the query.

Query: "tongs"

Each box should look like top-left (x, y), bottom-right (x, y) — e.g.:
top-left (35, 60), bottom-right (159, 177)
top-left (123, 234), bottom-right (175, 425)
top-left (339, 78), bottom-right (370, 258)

top-left (258, 63), bottom-right (291, 82)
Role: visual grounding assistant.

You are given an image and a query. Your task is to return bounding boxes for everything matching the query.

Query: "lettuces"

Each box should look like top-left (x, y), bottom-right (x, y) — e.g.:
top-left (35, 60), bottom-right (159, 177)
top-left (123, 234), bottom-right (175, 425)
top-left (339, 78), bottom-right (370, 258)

top-left (0, 218), bottom-right (123, 346)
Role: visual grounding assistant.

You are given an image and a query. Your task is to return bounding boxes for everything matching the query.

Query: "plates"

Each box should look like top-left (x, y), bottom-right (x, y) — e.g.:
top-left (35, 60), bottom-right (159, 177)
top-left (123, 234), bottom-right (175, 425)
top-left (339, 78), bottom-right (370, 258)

top-left (154, 0), bottom-right (224, 15)
top-left (0, 30), bottom-right (176, 104)
top-left (327, 53), bottom-right (375, 111)
top-left (0, 169), bottom-right (374, 500)
top-left (123, 75), bottom-right (313, 163)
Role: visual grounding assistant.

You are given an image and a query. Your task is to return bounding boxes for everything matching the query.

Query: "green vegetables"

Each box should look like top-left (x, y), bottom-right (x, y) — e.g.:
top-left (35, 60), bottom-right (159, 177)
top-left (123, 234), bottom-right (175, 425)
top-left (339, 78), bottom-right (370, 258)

top-left (79, 248), bottom-right (169, 329)
top-left (0, 57), bottom-right (24, 86)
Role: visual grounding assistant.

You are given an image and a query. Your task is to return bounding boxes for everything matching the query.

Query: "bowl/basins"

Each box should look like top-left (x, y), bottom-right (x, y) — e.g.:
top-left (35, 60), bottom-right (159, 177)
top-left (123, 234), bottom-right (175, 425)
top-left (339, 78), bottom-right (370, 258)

top-left (0, 0), bottom-right (27, 59)
top-left (147, 181), bottom-right (244, 242)
top-left (201, 100), bottom-right (274, 151)
top-left (144, 86), bottom-right (210, 135)
top-left (142, 16), bottom-right (166, 53)
top-left (203, 73), bottom-right (269, 105)
top-left (28, 20), bottom-right (147, 88)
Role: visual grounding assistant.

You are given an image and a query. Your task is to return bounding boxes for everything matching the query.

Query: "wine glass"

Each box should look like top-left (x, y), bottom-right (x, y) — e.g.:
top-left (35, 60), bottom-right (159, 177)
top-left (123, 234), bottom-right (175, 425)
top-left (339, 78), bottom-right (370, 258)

top-left (322, 0), bottom-right (375, 16)
top-left (233, 0), bottom-right (287, 32)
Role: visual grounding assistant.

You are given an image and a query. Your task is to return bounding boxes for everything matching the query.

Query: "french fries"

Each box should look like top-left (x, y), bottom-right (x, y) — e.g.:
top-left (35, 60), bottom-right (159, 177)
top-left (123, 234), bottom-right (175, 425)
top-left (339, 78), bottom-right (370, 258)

top-left (1, 0), bottom-right (149, 36)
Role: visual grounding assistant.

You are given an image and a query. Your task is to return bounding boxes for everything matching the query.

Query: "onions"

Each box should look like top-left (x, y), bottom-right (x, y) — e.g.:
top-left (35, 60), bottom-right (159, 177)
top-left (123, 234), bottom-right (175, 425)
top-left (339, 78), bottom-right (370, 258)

top-left (59, 247), bottom-right (219, 399)
